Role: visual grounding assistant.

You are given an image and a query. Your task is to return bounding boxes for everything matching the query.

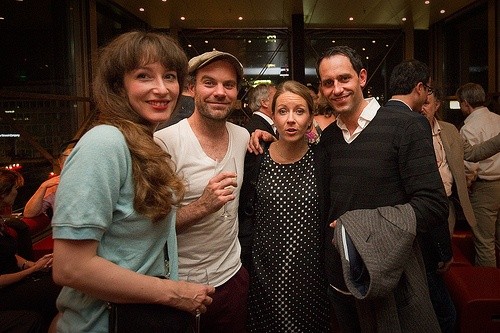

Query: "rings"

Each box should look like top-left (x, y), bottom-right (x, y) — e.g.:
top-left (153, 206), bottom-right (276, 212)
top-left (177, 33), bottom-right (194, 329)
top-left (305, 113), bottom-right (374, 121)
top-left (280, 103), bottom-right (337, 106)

top-left (194, 310), bottom-right (201, 316)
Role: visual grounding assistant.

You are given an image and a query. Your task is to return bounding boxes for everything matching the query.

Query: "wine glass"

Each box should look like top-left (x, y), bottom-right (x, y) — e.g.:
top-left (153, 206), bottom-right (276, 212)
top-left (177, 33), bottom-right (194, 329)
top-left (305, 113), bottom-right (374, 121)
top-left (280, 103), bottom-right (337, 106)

top-left (210, 157), bottom-right (237, 221)
top-left (187, 267), bottom-right (209, 333)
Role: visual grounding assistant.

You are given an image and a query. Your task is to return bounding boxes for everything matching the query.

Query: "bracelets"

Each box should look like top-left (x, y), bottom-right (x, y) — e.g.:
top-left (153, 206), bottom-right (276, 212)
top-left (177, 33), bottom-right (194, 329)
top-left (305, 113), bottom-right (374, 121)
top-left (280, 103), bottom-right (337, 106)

top-left (21, 261), bottom-right (29, 271)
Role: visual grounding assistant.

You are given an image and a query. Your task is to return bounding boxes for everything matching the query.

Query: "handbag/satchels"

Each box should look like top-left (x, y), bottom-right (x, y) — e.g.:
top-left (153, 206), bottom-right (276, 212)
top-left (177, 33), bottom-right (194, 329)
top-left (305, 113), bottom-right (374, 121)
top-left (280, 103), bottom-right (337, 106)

top-left (107, 297), bottom-right (200, 333)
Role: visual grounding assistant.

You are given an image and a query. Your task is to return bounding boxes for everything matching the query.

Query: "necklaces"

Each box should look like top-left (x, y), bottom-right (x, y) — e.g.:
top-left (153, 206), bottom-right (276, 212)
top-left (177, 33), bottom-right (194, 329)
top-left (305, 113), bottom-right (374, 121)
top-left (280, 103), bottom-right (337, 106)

top-left (275, 139), bottom-right (306, 161)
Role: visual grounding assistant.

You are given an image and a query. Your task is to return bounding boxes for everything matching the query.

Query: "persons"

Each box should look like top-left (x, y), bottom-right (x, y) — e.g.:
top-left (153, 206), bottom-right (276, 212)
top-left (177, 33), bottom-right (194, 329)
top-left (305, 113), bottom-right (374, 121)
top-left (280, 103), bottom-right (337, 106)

top-left (110, 45), bottom-right (253, 333)
top-left (48, 25), bottom-right (216, 333)
top-left (237, 45), bottom-right (500, 333)
top-left (0, 138), bottom-right (85, 333)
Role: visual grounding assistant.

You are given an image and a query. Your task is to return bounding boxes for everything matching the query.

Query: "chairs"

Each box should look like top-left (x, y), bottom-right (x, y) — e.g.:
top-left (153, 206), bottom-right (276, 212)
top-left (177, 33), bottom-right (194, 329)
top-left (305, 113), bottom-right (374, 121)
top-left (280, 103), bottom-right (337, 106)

top-left (4, 213), bottom-right (54, 286)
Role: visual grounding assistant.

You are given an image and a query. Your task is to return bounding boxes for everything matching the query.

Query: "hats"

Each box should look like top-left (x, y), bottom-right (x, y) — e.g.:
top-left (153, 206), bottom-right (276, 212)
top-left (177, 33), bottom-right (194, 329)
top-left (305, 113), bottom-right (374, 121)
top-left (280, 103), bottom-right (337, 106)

top-left (188, 50), bottom-right (244, 79)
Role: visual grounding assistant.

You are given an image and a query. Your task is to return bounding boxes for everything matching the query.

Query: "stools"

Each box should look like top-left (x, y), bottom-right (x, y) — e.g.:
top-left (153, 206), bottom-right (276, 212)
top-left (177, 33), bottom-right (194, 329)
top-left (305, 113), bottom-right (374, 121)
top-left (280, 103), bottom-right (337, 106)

top-left (443, 232), bottom-right (500, 333)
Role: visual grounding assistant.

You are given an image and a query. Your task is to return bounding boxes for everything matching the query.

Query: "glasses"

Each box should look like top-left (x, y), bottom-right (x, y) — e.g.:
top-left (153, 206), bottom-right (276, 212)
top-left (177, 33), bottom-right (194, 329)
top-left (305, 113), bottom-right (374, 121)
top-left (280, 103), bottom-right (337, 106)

top-left (422, 82), bottom-right (434, 95)
top-left (434, 141), bottom-right (444, 168)
